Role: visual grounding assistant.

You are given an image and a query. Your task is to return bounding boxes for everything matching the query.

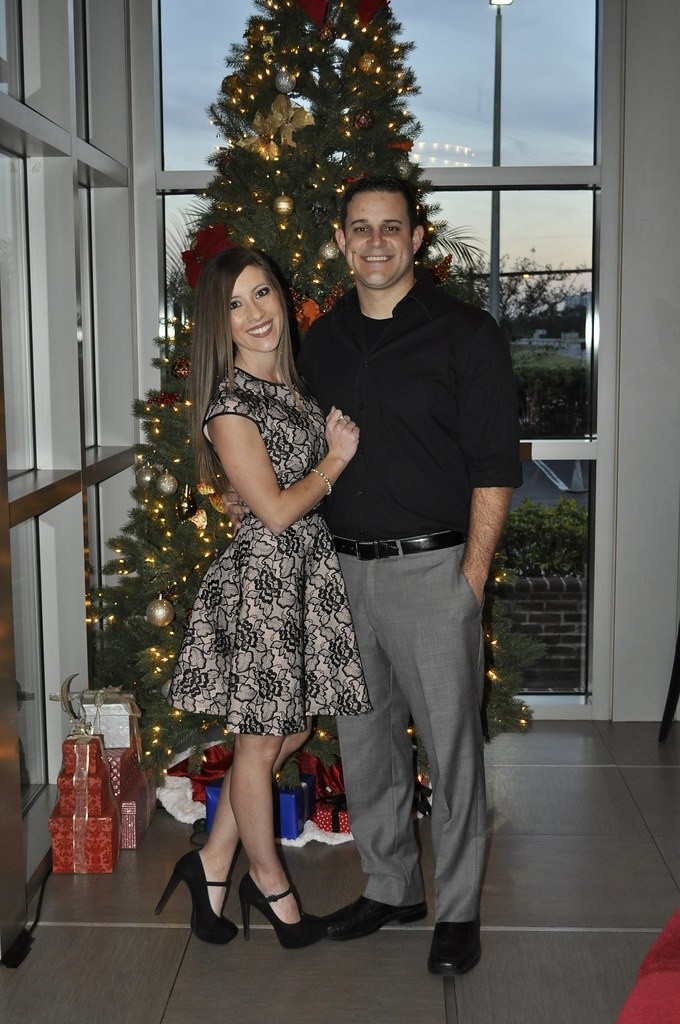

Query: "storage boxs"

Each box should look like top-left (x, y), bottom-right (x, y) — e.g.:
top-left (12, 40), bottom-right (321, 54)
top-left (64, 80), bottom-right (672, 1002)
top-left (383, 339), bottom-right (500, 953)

top-left (205, 774), bottom-right (314, 839)
top-left (63, 734), bottom-right (105, 773)
top-left (104, 734), bottom-right (143, 795)
top-left (58, 763), bottom-right (111, 815)
top-left (82, 701), bottom-right (133, 748)
top-left (338, 811), bottom-right (350, 833)
top-left (49, 795), bottom-right (126, 875)
top-left (314, 804), bottom-right (336, 832)
top-left (120, 770), bottom-right (157, 850)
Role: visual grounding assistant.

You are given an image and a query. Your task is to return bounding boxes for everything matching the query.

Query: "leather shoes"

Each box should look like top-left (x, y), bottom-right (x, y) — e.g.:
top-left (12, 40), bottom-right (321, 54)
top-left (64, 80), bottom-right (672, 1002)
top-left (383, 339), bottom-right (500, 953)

top-left (427, 922), bottom-right (482, 975)
top-left (319, 895), bottom-right (428, 938)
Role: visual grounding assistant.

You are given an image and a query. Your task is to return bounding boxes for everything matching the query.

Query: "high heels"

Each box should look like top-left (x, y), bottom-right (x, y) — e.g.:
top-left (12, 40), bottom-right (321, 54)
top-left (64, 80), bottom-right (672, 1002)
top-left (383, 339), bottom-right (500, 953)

top-left (239, 870), bottom-right (330, 949)
top-left (154, 850), bottom-right (238, 945)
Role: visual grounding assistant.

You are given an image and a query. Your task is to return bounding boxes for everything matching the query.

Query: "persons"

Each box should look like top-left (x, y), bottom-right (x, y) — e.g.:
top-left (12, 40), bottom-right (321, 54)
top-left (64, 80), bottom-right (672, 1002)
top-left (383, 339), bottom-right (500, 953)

top-left (220, 176), bottom-right (522, 974)
top-left (154, 246), bottom-right (374, 950)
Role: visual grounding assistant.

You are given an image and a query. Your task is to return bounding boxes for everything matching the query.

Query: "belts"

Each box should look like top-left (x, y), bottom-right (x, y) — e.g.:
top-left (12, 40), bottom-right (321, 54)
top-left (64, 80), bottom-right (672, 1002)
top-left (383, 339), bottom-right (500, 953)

top-left (328, 530), bottom-right (466, 557)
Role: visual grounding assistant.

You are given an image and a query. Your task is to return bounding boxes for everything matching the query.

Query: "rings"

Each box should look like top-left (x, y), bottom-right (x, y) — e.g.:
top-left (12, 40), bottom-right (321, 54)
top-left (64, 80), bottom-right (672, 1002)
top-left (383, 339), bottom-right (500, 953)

top-left (338, 416), bottom-right (350, 425)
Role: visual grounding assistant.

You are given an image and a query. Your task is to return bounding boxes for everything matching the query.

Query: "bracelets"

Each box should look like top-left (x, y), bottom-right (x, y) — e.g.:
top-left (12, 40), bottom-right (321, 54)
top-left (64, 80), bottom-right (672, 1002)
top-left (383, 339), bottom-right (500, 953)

top-left (311, 468), bottom-right (331, 495)
